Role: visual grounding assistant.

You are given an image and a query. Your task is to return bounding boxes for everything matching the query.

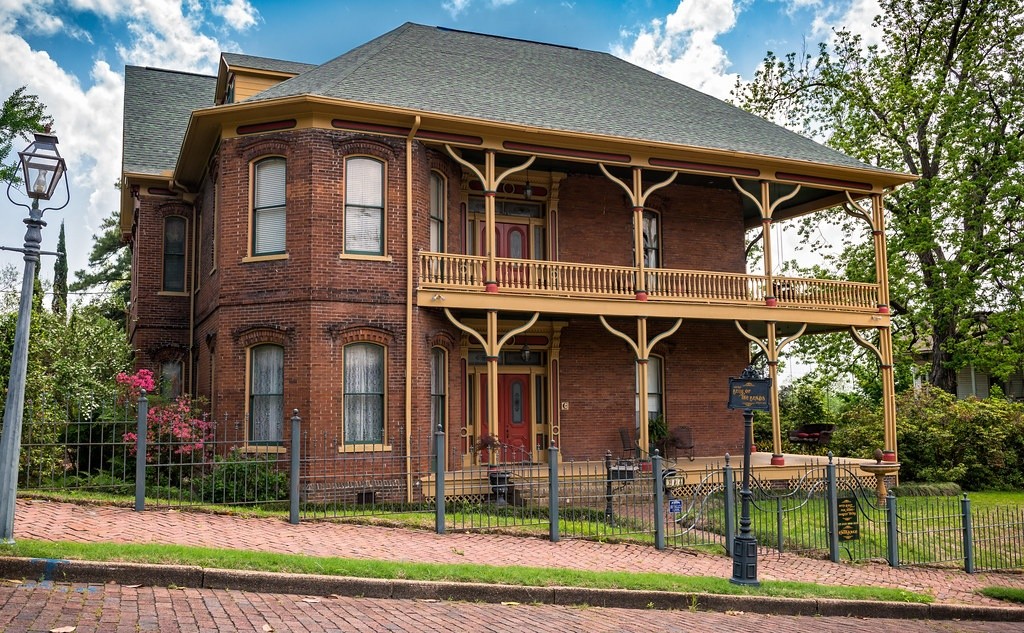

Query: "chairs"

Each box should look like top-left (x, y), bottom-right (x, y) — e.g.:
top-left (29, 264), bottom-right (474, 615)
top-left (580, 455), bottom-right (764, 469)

top-left (618, 427), bottom-right (639, 459)
top-left (671, 426), bottom-right (695, 462)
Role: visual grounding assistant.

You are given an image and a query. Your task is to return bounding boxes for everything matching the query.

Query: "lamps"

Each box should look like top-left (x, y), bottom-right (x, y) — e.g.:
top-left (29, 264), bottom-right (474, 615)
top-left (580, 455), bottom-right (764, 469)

top-left (520, 316), bottom-right (530, 362)
top-left (524, 167), bottom-right (532, 200)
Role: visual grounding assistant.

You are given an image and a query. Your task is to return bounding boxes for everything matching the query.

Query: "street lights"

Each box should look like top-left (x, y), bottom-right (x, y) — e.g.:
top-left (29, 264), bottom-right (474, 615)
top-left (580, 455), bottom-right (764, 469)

top-left (0, 123), bottom-right (70, 546)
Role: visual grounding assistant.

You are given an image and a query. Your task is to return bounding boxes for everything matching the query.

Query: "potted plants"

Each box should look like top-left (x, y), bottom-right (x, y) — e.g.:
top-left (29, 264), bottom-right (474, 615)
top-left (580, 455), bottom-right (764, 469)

top-left (643, 416), bottom-right (669, 457)
